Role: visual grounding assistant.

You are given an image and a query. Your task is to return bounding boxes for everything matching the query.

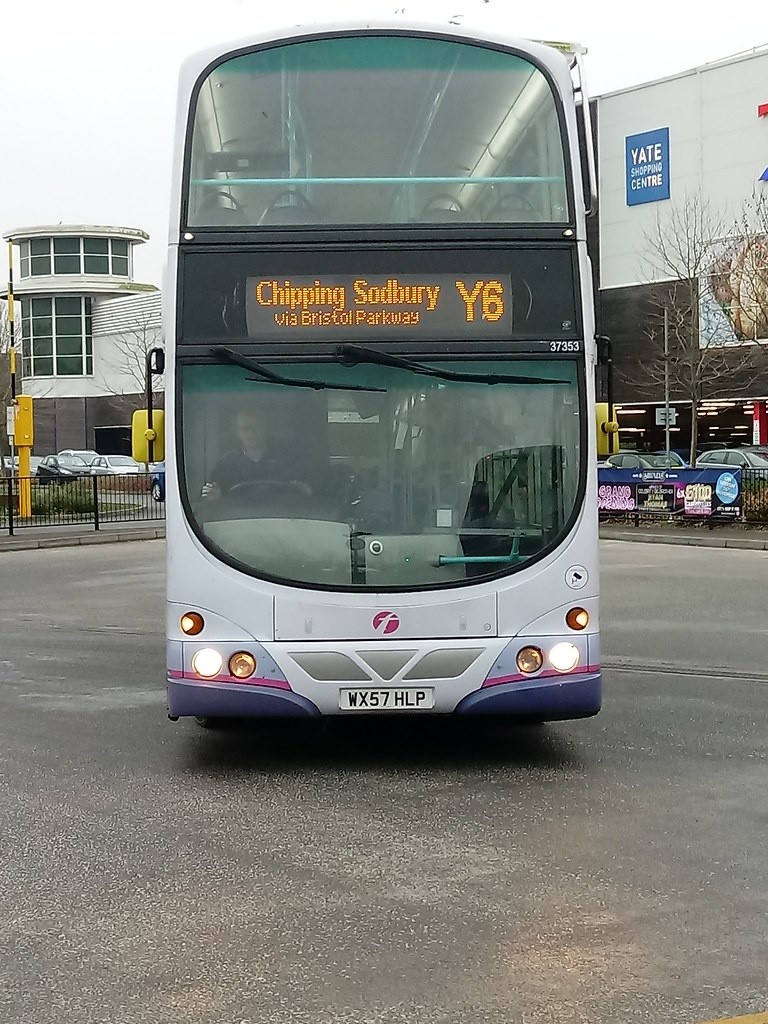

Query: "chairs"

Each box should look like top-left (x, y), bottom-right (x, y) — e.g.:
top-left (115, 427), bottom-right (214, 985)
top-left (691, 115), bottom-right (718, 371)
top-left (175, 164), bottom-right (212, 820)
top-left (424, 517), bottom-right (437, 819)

top-left (226, 444), bottom-right (491, 530)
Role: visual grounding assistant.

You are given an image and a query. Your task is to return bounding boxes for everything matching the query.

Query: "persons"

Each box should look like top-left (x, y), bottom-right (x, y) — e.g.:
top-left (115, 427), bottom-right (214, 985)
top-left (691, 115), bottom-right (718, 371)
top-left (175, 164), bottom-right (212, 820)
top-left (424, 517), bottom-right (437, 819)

top-left (201, 404), bottom-right (314, 504)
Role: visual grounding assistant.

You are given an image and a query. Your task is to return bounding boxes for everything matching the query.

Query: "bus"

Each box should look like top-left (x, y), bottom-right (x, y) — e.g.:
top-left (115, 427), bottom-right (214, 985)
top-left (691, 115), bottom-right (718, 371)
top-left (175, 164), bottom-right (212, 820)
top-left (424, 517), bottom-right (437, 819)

top-left (131, 16), bottom-right (619, 730)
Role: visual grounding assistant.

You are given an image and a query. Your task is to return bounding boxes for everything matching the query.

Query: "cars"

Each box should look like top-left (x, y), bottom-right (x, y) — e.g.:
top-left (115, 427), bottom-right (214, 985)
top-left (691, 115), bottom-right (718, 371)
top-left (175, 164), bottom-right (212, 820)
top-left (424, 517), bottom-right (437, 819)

top-left (596, 443), bottom-right (768, 487)
top-left (1, 447), bottom-right (166, 503)
